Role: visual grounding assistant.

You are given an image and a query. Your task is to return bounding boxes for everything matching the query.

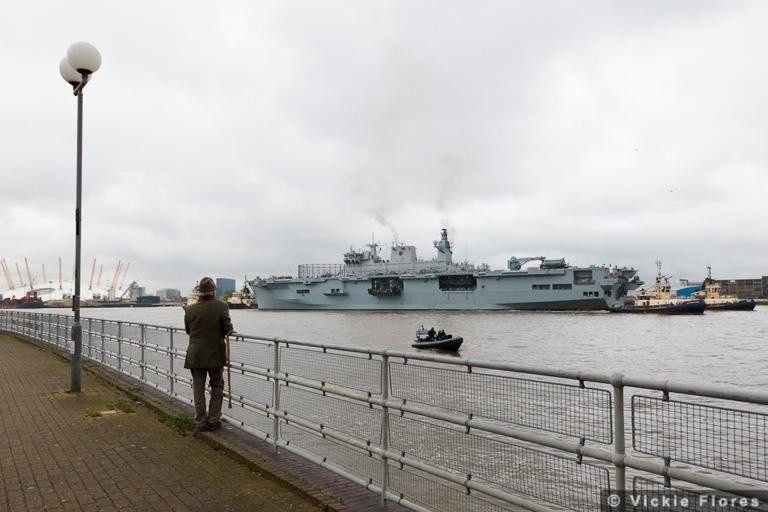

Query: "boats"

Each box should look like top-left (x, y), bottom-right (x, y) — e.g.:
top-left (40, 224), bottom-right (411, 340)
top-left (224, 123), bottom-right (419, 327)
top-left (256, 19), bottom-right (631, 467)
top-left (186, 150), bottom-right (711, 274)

top-left (411, 323), bottom-right (463, 351)
top-left (607, 257), bottom-right (756, 313)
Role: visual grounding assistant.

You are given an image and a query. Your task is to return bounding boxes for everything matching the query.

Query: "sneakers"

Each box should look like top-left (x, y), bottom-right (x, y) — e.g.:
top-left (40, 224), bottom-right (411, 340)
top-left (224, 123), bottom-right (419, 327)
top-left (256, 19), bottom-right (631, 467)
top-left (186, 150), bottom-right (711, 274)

top-left (196, 421), bottom-right (221, 432)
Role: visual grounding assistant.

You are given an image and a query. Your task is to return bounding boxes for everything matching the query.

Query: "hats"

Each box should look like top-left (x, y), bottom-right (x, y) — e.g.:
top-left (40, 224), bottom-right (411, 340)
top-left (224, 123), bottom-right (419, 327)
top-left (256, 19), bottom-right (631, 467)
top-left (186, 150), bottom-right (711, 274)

top-left (195, 278), bottom-right (219, 292)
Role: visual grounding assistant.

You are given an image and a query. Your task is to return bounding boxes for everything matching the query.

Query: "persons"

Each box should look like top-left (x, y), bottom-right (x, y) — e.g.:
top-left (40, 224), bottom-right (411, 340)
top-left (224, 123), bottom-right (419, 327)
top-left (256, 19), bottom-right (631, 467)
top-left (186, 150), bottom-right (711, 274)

top-left (183, 277), bottom-right (233, 430)
top-left (427, 327), bottom-right (446, 342)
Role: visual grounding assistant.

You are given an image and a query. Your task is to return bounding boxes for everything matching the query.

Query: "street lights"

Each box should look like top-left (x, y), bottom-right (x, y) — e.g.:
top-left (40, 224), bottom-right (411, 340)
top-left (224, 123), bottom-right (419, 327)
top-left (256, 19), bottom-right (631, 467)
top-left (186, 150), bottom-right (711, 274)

top-left (59, 42), bottom-right (102, 392)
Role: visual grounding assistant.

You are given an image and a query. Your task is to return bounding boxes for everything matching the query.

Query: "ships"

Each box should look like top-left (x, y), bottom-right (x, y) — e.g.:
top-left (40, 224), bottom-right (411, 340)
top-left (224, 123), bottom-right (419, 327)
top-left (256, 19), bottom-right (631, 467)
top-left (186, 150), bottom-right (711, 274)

top-left (244, 228), bottom-right (645, 310)
